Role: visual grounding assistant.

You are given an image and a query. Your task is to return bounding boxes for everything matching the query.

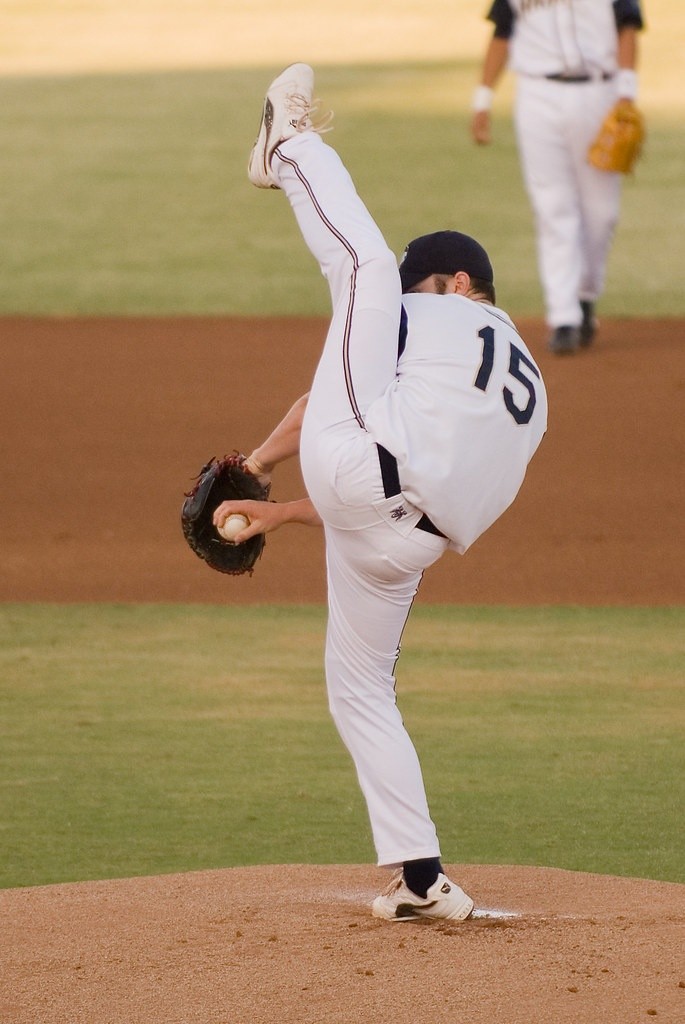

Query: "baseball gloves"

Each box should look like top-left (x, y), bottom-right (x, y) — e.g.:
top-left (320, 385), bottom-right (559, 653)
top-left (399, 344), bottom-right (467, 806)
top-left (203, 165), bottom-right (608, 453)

top-left (584, 97), bottom-right (647, 174)
top-left (181, 455), bottom-right (271, 576)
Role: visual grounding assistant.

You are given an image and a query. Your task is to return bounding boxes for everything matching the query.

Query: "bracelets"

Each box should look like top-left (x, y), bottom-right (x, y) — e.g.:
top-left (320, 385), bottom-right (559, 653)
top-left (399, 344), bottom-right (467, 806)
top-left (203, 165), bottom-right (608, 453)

top-left (615, 68), bottom-right (638, 100)
top-left (472, 85), bottom-right (494, 114)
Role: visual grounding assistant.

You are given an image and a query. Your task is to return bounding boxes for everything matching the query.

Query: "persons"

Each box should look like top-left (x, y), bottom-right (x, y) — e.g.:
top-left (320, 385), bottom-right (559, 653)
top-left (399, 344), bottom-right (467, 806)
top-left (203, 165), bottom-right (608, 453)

top-left (472, 0), bottom-right (645, 353)
top-left (213, 64), bottom-right (548, 922)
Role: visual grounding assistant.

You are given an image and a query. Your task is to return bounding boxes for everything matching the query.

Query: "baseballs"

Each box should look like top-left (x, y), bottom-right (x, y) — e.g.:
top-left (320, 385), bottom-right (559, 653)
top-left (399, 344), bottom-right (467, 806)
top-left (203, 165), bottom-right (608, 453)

top-left (217, 513), bottom-right (250, 541)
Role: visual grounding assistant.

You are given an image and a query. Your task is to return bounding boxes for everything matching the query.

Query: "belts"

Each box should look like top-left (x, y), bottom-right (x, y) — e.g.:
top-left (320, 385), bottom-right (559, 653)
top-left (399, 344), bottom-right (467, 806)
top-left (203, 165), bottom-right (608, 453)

top-left (377, 443), bottom-right (448, 538)
top-left (546, 73), bottom-right (608, 81)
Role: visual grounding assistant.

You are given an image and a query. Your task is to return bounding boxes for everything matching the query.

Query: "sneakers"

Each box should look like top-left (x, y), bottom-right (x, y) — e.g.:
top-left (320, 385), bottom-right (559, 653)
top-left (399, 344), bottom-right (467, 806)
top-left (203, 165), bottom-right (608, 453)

top-left (372, 867), bottom-right (474, 921)
top-left (247, 62), bottom-right (335, 189)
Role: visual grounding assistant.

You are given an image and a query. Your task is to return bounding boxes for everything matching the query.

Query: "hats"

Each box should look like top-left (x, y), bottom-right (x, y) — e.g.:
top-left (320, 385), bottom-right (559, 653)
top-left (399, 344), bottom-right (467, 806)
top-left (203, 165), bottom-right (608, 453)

top-left (399, 230), bottom-right (493, 294)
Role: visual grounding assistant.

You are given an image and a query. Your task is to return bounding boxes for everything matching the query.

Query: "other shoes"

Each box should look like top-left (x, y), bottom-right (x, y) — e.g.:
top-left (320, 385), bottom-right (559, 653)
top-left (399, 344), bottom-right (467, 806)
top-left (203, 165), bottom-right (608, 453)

top-left (581, 301), bottom-right (594, 334)
top-left (553, 327), bottom-right (575, 353)
